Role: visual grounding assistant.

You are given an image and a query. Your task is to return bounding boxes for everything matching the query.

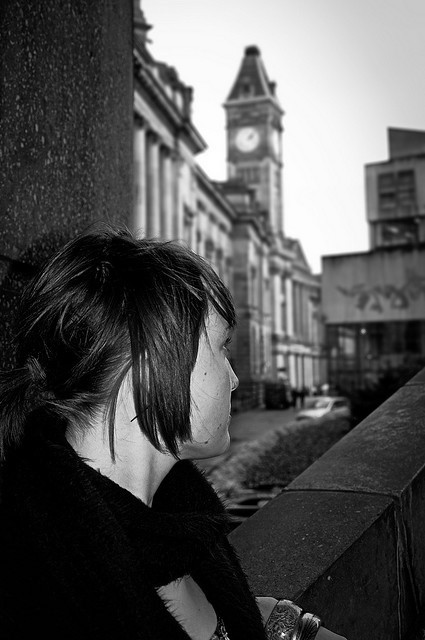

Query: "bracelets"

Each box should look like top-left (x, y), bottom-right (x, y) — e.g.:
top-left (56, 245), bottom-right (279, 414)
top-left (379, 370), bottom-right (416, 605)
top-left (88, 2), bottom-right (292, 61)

top-left (291, 613), bottom-right (320, 640)
top-left (264, 599), bottom-right (302, 640)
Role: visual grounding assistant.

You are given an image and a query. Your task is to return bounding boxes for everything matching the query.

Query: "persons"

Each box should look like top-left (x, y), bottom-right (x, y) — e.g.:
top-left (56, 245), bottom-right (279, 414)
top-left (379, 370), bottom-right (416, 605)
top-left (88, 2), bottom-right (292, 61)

top-left (0, 229), bottom-right (348, 640)
top-left (291, 388), bottom-right (298, 409)
top-left (299, 389), bottom-right (305, 408)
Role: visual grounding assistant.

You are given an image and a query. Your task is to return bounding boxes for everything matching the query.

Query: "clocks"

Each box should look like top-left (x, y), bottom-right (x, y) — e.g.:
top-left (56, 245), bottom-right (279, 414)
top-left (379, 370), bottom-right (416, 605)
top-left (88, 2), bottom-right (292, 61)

top-left (233, 126), bottom-right (259, 154)
